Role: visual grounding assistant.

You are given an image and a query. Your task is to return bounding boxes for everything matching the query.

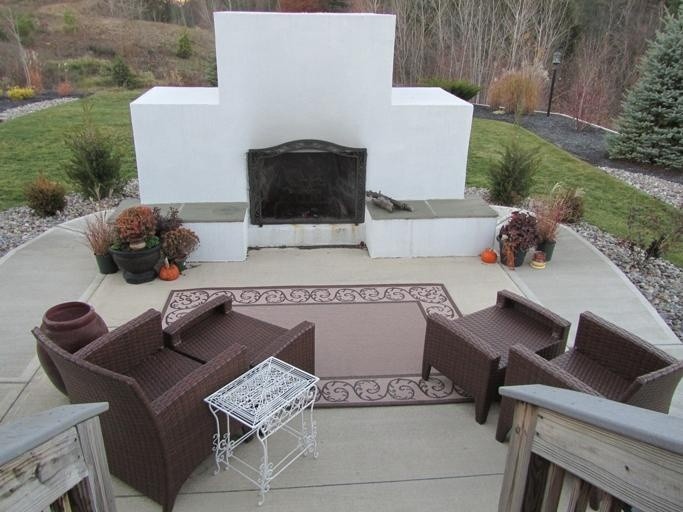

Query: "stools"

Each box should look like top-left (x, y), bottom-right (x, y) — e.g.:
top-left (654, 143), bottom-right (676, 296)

top-left (162, 295), bottom-right (316, 401)
top-left (422, 287), bottom-right (572, 425)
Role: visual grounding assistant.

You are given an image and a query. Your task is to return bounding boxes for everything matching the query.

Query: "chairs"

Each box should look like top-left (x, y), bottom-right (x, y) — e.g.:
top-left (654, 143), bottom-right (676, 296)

top-left (31, 308), bottom-right (250, 511)
top-left (496, 310), bottom-right (683, 444)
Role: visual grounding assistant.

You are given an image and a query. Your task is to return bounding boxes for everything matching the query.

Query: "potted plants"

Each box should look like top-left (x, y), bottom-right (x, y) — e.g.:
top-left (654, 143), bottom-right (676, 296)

top-left (78, 204), bottom-right (201, 282)
top-left (496, 190), bottom-right (573, 267)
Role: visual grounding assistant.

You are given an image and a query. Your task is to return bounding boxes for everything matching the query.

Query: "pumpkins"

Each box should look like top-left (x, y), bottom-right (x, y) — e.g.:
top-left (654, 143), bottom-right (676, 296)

top-left (159, 258), bottom-right (179, 281)
top-left (481, 248), bottom-right (497, 264)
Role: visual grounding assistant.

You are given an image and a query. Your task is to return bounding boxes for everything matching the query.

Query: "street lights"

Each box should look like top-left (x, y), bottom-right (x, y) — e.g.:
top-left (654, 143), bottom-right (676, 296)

top-left (546, 51), bottom-right (562, 117)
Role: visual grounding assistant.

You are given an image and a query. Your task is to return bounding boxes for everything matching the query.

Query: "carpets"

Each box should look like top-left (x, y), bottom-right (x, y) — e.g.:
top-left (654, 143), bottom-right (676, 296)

top-left (160, 282), bottom-right (479, 407)
